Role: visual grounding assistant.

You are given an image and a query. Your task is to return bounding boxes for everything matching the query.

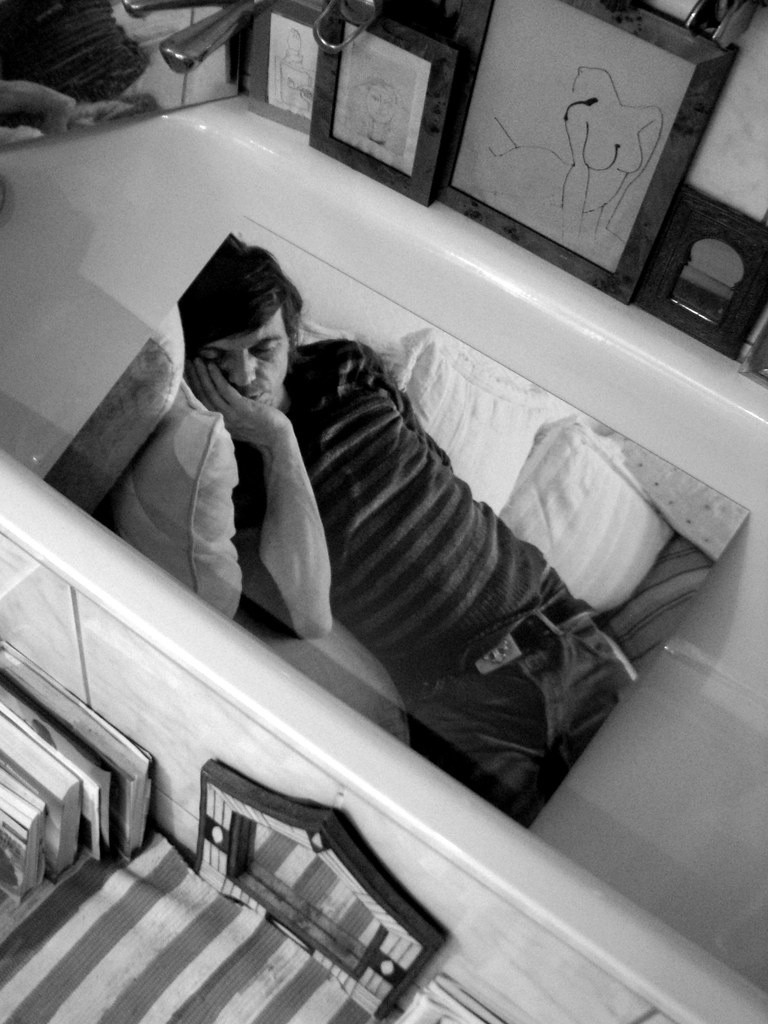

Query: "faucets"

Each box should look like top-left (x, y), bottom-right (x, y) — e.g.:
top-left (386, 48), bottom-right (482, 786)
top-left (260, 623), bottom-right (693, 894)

top-left (159, 1), bottom-right (287, 75)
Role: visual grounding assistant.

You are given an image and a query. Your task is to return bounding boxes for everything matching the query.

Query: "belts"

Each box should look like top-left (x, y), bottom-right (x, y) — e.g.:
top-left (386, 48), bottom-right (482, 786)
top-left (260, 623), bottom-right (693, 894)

top-left (463, 599), bottom-right (596, 677)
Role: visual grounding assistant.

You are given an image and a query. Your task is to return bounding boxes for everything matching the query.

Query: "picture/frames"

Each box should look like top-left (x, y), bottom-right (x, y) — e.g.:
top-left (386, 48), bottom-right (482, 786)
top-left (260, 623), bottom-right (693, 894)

top-left (249, 0), bottom-right (321, 136)
top-left (435, 0), bottom-right (741, 306)
top-left (310, 2), bottom-right (466, 208)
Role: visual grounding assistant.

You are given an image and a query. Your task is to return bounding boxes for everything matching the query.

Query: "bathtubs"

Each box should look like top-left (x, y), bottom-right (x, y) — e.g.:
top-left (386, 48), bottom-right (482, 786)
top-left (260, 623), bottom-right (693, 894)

top-left (1, 92), bottom-right (768, 1022)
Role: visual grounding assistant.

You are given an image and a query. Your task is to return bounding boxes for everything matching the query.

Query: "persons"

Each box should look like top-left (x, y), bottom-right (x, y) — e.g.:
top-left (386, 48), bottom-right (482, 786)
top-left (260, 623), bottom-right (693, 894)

top-left (177, 245), bottom-right (640, 829)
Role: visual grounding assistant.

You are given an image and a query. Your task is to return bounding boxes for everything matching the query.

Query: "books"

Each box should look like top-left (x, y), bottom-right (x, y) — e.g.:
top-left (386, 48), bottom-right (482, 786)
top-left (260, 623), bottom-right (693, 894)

top-left (0, 639), bottom-right (155, 905)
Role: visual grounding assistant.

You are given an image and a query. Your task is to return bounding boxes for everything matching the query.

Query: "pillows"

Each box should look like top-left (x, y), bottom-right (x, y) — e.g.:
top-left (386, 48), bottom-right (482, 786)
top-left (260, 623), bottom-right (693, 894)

top-left (587, 531), bottom-right (714, 673)
top-left (42, 332), bottom-right (183, 518)
top-left (404, 340), bottom-right (546, 517)
top-left (99, 377), bottom-right (243, 620)
top-left (499, 415), bottom-right (674, 619)
